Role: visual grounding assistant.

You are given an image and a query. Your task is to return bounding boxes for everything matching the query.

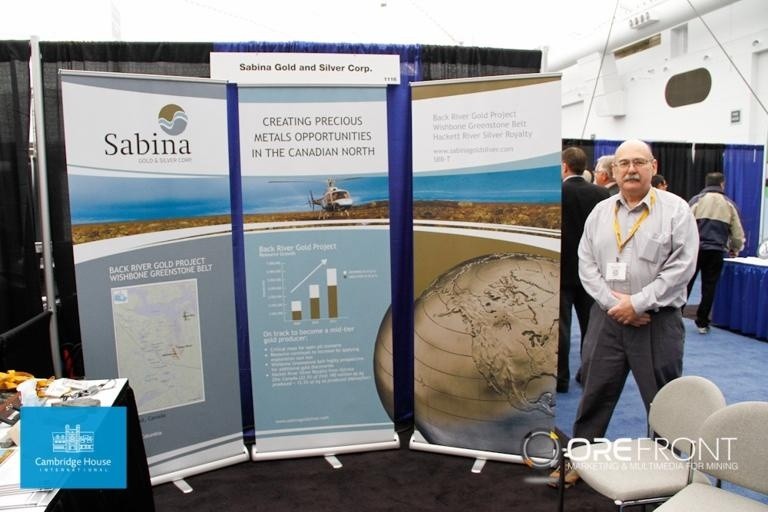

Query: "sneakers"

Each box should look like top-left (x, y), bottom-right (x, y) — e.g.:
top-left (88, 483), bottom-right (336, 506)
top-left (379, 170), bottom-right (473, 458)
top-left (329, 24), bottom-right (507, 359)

top-left (556, 375), bottom-right (581, 393)
top-left (697, 326), bottom-right (711, 334)
top-left (545, 463), bottom-right (580, 488)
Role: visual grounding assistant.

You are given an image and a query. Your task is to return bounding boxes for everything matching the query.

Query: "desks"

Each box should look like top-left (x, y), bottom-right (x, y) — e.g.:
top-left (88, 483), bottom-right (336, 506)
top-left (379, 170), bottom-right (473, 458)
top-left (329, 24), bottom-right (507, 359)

top-left (709, 252), bottom-right (768, 341)
top-left (0, 375), bottom-right (156, 512)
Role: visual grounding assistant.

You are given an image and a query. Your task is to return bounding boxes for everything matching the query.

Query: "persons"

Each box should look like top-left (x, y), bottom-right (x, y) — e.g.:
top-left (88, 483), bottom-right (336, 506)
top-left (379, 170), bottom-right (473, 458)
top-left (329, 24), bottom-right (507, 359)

top-left (594, 154), bottom-right (619, 196)
top-left (650, 174), bottom-right (668, 190)
top-left (680, 171), bottom-right (746, 334)
top-left (543, 139), bottom-right (699, 488)
top-left (555, 145), bottom-right (612, 393)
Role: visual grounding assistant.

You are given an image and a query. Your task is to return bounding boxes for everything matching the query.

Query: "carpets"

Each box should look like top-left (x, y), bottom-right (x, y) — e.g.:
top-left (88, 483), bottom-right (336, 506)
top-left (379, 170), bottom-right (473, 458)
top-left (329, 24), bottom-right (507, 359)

top-left (146, 427), bottom-right (664, 512)
top-left (681, 302), bottom-right (713, 326)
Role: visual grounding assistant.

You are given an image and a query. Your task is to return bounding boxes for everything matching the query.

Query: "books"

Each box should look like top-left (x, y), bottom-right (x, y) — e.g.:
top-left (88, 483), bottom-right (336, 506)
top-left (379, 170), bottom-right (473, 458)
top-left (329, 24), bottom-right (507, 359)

top-left (0, 447), bottom-right (53, 509)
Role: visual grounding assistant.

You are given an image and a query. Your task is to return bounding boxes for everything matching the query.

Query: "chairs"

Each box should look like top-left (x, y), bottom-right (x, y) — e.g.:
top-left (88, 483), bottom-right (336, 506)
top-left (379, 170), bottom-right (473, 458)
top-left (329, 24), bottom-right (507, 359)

top-left (522, 375), bottom-right (768, 512)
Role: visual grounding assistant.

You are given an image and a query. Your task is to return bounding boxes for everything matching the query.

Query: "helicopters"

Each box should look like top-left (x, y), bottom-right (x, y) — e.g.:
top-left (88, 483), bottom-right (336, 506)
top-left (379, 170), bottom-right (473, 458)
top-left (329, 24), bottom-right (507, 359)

top-left (266, 176), bottom-right (360, 212)
